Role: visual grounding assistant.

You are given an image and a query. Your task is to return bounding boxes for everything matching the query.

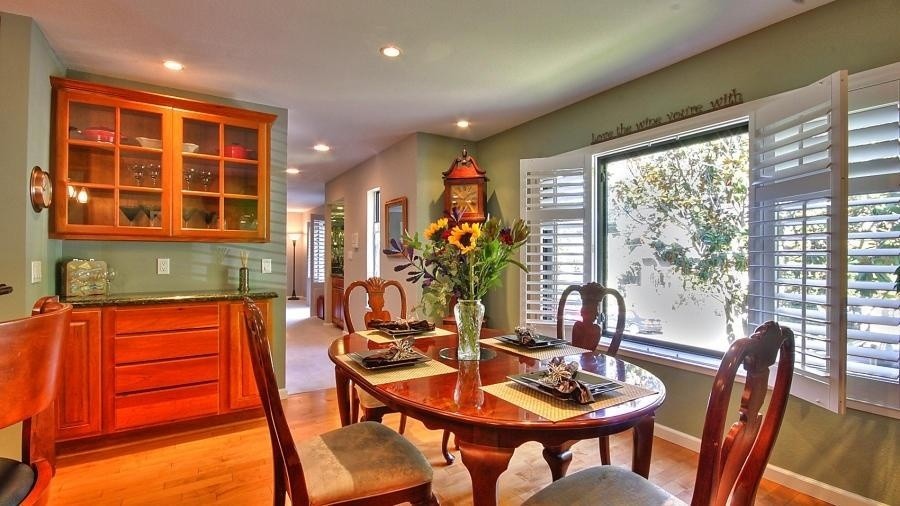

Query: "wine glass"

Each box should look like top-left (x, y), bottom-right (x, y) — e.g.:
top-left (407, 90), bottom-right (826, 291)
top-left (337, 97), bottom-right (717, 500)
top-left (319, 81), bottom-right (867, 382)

top-left (121, 204), bottom-right (141, 228)
top-left (199, 210), bottom-right (217, 230)
top-left (142, 205), bottom-right (162, 228)
top-left (181, 167), bottom-right (197, 190)
top-left (198, 169), bottom-right (217, 191)
top-left (148, 164), bottom-right (161, 185)
top-left (183, 206), bottom-right (198, 228)
top-left (129, 163), bottom-right (145, 187)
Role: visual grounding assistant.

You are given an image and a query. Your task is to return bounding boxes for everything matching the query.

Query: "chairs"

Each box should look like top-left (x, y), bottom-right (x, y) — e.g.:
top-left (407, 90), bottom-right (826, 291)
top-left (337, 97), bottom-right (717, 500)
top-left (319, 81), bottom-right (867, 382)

top-left (520, 321), bottom-right (796, 506)
top-left (0, 301), bottom-right (72, 506)
top-left (344, 276), bottom-right (407, 435)
top-left (557, 282), bottom-right (626, 464)
top-left (242, 297), bottom-right (433, 506)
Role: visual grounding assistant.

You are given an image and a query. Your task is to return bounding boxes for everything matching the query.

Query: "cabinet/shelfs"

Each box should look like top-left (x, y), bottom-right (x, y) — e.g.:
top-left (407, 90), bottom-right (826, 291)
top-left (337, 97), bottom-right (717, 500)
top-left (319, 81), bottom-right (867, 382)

top-left (113, 305), bottom-right (220, 430)
top-left (229, 302), bottom-right (268, 409)
top-left (332, 277), bottom-right (344, 327)
top-left (56, 310), bottom-right (102, 441)
top-left (53, 87), bottom-right (267, 239)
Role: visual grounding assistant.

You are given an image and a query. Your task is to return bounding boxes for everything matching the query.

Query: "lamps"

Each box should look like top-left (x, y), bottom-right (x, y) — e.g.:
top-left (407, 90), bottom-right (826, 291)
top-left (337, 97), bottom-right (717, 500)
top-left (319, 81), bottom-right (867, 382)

top-left (288, 233), bottom-right (300, 300)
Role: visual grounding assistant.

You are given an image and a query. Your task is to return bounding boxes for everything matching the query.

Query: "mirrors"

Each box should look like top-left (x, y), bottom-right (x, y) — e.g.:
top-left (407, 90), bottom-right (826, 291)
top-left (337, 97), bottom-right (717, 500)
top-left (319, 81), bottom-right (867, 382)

top-left (384, 196), bottom-right (409, 257)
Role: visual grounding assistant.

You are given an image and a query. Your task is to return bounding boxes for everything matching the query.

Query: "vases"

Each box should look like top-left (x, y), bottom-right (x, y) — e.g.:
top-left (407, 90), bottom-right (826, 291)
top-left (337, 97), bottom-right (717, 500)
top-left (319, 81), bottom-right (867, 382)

top-left (453, 298), bottom-right (485, 361)
top-left (454, 361), bottom-right (484, 411)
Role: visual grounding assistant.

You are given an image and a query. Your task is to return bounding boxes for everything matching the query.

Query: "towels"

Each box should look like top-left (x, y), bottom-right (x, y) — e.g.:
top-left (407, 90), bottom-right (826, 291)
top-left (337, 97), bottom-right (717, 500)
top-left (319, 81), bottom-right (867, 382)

top-left (368, 319), bottom-right (436, 333)
top-left (514, 324), bottom-right (541, 346)
top-left (364, 336), bottom-right (421, 365)
top-left (539, 356), bottom-right (595, 404)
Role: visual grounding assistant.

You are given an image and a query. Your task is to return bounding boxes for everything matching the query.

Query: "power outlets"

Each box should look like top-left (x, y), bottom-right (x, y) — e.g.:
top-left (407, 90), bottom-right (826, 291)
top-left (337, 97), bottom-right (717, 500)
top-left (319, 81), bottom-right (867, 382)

top-left (157, 258), bottom-right (169, 275)
top-left (31, 261), bottom-right (41, 284)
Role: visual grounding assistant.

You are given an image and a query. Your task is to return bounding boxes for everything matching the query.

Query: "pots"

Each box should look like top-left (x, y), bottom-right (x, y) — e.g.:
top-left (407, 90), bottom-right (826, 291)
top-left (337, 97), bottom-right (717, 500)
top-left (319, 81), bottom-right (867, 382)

top-left (218, 143), bottom-right (254, 160)
top-left (83, 129), bottom-right (126, 144)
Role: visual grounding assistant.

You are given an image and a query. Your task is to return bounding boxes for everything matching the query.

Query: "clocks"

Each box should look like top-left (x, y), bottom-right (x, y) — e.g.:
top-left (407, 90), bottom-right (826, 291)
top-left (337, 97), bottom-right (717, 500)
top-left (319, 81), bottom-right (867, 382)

top-left (441, 148), bottom-right (491, 228)
top-left (30, 166), bottom-right (52, 212)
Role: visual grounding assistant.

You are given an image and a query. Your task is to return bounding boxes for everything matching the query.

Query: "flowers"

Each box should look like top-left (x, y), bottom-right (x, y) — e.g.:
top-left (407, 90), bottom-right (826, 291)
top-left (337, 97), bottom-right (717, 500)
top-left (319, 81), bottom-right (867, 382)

top-left (383, 207), bottom-right (531, 350)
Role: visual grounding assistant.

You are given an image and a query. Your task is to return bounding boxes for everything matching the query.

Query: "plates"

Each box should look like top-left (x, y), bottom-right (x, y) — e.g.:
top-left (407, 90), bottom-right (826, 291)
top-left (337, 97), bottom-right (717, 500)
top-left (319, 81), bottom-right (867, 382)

top-left (354, 349), bottom-right (422, 362)
top-left (505, 373), bottom-right (625, 401)
top-left (346, 350), bottom-right (433, 370)
top-left (137, 136), bottom-right (163, 149)
top-left (496, 336), bottom-right (570, 350)
top-left (378, 329), bottom-right (430, 335)
top-left (180, 143), bottom-right (200, 152)
top-left (520, 372), bottom-right (614, 391)
top-left (500, 334), bottom-right (558, 344)
